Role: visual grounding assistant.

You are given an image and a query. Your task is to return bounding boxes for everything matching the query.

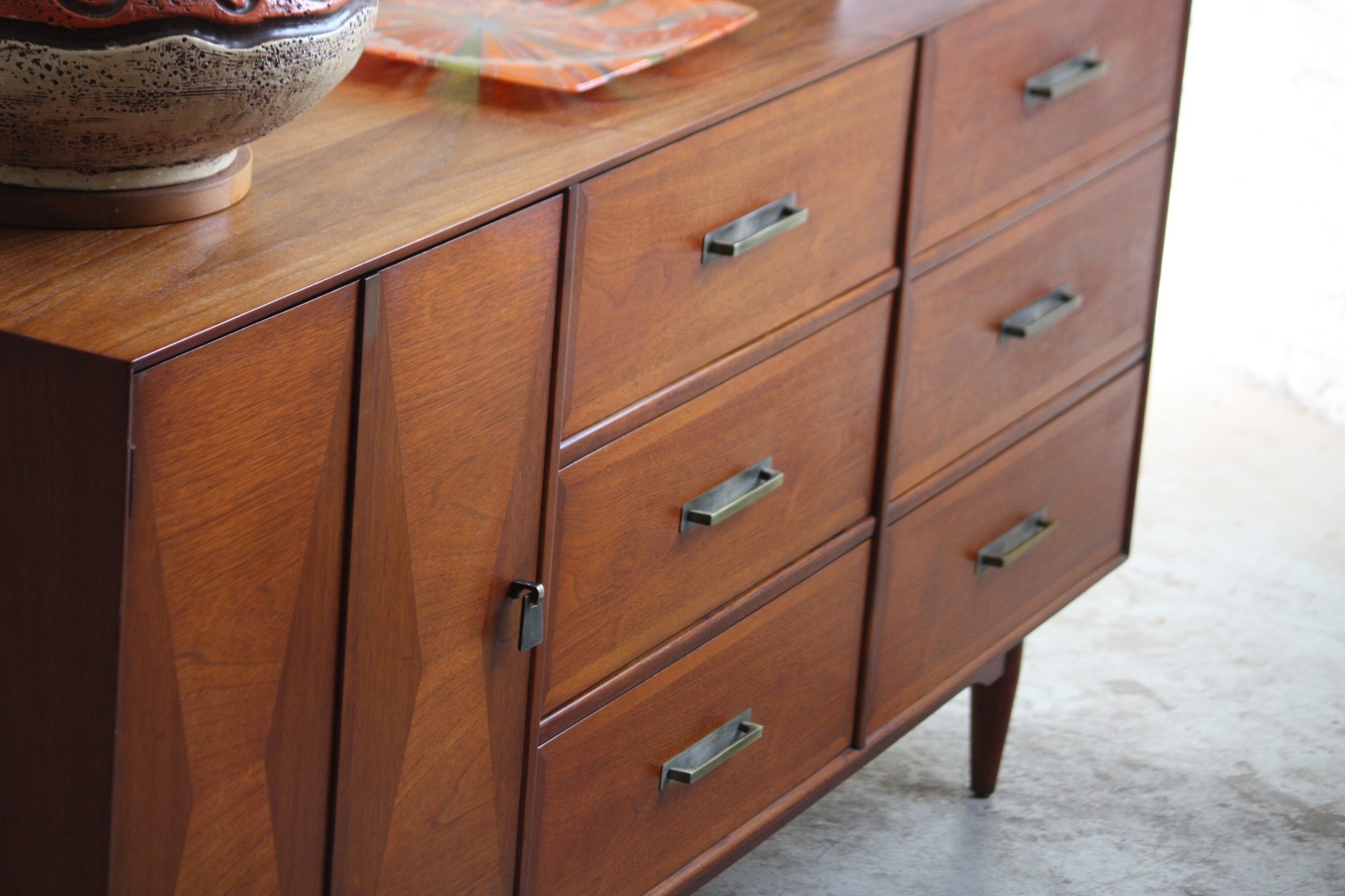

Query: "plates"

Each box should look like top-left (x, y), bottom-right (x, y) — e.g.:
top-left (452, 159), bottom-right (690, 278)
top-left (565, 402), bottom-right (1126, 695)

top-left (364, 0), bottom-right (761, 92)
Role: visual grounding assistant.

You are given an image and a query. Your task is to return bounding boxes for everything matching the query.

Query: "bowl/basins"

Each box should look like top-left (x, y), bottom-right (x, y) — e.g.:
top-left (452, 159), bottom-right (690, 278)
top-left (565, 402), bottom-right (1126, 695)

top-left (0, 0), bottom-right (380, 229)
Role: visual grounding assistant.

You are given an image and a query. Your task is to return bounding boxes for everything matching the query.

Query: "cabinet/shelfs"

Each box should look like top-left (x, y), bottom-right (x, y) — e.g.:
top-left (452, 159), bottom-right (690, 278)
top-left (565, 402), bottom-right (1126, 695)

top-left (0, 0), bottom-right (1190, 894)
